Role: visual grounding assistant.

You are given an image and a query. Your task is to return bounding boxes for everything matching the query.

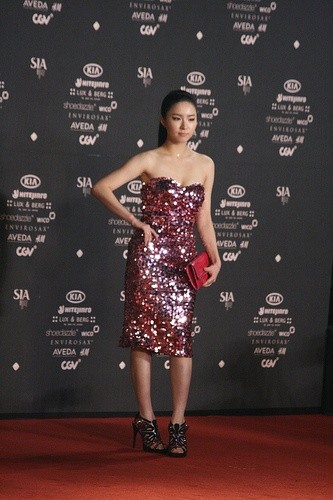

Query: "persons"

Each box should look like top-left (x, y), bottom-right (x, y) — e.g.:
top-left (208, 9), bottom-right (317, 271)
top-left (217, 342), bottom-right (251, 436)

top-left (91, 90), bottom-right (221, 458)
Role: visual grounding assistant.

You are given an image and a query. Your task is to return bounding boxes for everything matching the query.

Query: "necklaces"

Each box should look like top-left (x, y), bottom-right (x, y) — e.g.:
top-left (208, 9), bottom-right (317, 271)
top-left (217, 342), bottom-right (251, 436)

top-left (166, 144), bottom-right (186, 157)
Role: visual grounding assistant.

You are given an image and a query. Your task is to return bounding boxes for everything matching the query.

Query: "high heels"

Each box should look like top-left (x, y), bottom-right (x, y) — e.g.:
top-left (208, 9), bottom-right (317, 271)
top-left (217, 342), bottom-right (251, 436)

top-left (132, 411), bottom-right (167, 453)
top-left (167, 422), bottom-right (189, 456)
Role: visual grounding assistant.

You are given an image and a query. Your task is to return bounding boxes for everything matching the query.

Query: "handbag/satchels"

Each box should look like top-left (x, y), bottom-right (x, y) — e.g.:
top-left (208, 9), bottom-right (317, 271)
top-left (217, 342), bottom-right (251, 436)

top-left (185, 251), bottom-right (212, 289)
